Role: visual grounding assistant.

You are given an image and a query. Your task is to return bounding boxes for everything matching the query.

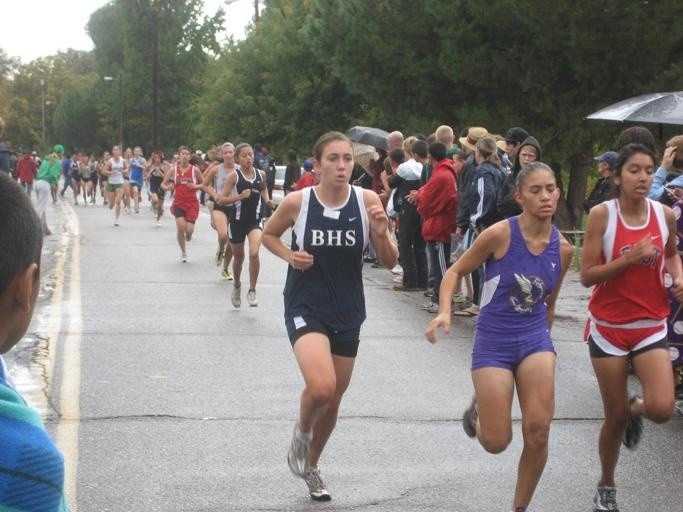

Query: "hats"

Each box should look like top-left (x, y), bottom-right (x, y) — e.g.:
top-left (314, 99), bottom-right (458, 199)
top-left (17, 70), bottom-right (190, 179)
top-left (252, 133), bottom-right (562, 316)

top-left (495, 127), bottom-right (530, 153)
top-left (593, 151), bottom-right (622, 165)
top-left (53, 144), bottom-right (64, 155)
top-left (459, 126), bottom-right (490, 152)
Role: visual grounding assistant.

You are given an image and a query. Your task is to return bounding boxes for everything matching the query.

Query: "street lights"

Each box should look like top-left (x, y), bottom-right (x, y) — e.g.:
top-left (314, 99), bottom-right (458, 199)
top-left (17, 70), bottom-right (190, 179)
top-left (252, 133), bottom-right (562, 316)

top-left (102, 70), bottom-right (126, 151)
top-left (39, 77), bottom-right (47, 150)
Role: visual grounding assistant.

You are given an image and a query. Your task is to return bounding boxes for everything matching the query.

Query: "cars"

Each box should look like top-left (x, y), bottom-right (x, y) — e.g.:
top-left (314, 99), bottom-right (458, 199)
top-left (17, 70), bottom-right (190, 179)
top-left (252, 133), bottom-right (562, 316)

top-left (270, 166), bottom-right (311, 206)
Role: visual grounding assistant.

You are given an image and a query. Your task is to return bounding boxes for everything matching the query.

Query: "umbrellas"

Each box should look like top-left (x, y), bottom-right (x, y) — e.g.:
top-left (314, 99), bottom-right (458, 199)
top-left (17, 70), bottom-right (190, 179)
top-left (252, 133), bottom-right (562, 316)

top-left (580, 89), bottom-right (682, 126)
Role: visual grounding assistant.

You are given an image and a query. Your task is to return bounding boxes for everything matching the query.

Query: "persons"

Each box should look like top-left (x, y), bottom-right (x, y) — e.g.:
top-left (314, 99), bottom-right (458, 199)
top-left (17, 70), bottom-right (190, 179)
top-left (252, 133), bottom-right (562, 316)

top-left (0, 170), bottom-right (69, 512)
top-left (102, 144), bottom-right (167, 226)
top-left (584, 127), bottom-right (683, 413)
top-left (202, 142), bottom-right (275, 307)
top-left (284, 150), bottom-right (315, 201)
top-left (58, 147), bottom-right (112, 205)
top-left (421, 161), bottom-right (575, 511)
top-left (160, 144), bottom-right (204, 261)
top-left (0, 144), bottom-right (66, 204)
top-left (578, 141), bottom-right (683, 512)
top-left (343, 126), bottom-right (541, 315)
top-left (261, 130), bottom-right (401, 501)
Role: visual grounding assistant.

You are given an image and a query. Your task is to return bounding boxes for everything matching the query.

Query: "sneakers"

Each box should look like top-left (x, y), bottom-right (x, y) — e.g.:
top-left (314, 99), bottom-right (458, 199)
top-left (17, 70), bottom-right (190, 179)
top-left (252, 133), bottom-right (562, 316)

top-left (42, 224), bottom-right (52, 236)
top-left (621, 388), bottom-right (645, 450)
top-left (181, 252), bottom-right (188, 263)
top-left (230, 282), bottom-right (242, 308)
top-left (214, 247), bottom-right (226, 266)
top-left (287, 421), bottom-right (314, 479)
top-left (303, 464), bottom-right (333, 502)
top-left (247, 289), bottom-right (259, 308)
top-left (53, 190), bottom-right (165, 227)
top-left (592, 482), bottom-right (620, 512)
top-left (363, 254), bottom-right (482, 316)
top-left (462, 393), bottom-right (484, 439)
top-left (184, 232), bottom-right (192, 242)
top-left (221, 268), bottom-right (233, 281)
